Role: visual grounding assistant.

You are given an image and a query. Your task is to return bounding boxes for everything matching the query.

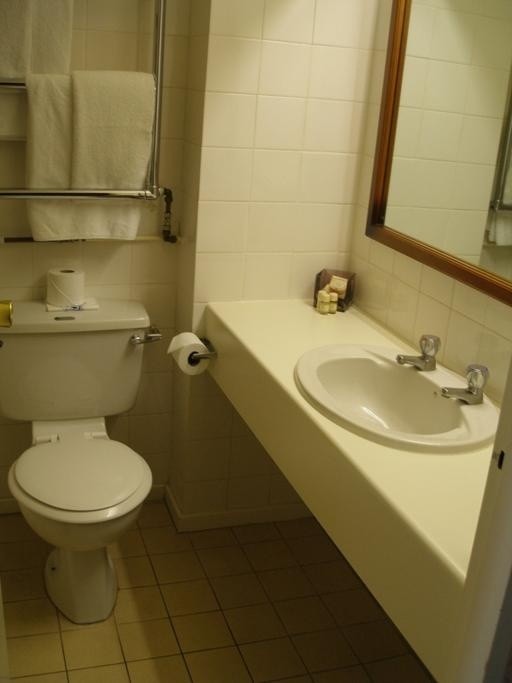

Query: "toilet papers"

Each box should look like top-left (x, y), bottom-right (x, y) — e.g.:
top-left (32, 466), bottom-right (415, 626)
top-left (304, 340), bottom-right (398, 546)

top-left (45, 265), bottom-right (87, 308)
top-left (165, 331), bottom-right (211, 376)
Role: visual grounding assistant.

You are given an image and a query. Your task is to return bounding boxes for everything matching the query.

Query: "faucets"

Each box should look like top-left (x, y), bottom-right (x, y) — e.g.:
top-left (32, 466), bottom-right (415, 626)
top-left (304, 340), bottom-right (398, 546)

top-left (397, 334), bottom-right (441, 371)
top-left (441, 364), bottom-right (490, 405)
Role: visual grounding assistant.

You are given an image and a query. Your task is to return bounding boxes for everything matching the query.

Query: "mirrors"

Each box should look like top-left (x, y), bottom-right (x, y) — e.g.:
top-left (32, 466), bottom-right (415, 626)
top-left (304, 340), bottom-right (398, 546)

top-left (363, 0), bottom-right (512, 308)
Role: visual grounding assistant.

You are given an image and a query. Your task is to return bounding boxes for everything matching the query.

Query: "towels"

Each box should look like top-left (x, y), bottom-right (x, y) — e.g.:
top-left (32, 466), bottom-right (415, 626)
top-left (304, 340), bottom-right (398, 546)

top-left (71, 69), bottom-right (157, 190)
top-left (23, 72), bottom-right (142, 241)
top-left (489, 155), bottom-right (512, 246)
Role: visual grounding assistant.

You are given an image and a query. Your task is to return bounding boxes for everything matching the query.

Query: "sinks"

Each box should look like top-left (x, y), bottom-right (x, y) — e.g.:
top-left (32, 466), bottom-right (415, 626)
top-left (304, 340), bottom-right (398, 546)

top-left (293, 343), bottom-right (501, 455)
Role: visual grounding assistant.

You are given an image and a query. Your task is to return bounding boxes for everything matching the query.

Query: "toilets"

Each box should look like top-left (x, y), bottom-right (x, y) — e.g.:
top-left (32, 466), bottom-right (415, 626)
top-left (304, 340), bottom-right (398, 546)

top-left (0, 296), bottom-right (154, 625)
